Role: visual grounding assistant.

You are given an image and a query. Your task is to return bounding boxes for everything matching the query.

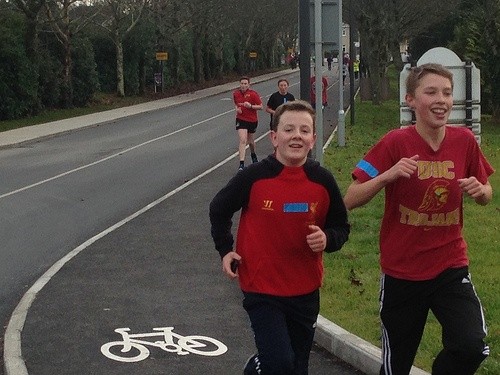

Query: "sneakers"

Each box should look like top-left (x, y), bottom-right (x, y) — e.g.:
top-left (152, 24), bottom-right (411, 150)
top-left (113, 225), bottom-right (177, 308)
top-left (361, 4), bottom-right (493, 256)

top-left (251, 153), bottom-right (259, 163)
top-left (237, 353), bottom-right (274, 375)
top-left (239, 164), bottom-right (244, 170)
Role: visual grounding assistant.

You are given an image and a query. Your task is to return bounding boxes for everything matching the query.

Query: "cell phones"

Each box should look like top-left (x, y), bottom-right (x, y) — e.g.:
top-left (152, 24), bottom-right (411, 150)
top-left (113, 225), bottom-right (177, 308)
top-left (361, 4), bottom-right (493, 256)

top-left (231, 261), bottom-right (238, 273)
